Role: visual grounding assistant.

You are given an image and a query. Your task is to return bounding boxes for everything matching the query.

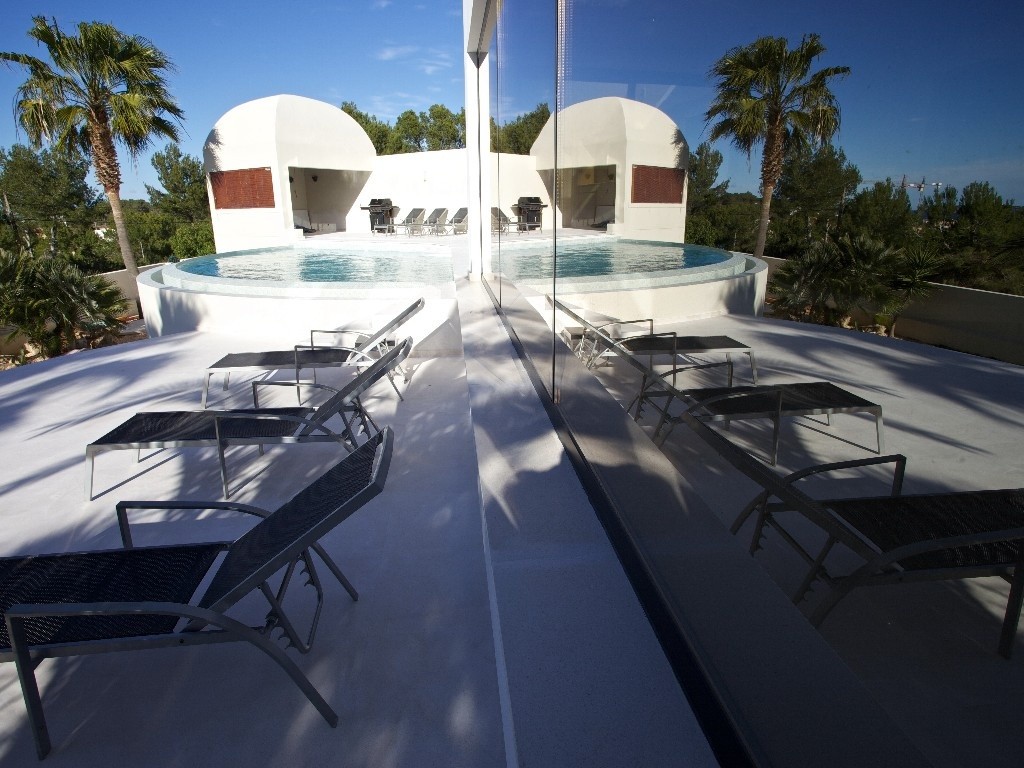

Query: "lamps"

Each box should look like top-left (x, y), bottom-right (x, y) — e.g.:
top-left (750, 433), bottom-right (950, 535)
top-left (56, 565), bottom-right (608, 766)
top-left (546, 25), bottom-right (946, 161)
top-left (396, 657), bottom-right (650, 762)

top-left (289, 177), bottom-right (294, 183)
top-left (311, 176), bottom-right (318, 182)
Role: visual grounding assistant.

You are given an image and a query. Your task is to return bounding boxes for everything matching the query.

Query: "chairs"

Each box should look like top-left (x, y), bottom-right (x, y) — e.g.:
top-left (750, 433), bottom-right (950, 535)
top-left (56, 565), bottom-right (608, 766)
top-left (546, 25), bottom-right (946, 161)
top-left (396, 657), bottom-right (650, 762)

top-left (0, 425), bottom-right (395, 758)
top-left (587, 329), bottom-right (885, 467)
top-left (490, 207), bottom-right (530, 236)
top-left (546, 295), bottom-right (759, 387)
top-left (436, 208), bottom-right (468, 235)
top-left (200, 297), bottom-right (425, 408)
top-left (679, 413), bottom-right (1024, 659)
top-left (84, 336), bottom-right (414, 503)
top-left (373, 209), bottom-right (425, 236)
top-left (407, 208), bottom-right (447, 237)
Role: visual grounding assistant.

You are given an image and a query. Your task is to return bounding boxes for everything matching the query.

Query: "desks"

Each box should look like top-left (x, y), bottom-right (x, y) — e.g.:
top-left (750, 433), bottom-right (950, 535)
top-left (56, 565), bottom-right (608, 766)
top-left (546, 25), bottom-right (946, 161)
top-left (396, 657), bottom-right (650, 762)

top-left (361, 206), bottom-right (397, 233)
top-left (512, 204), bottom-right (548, 235)
